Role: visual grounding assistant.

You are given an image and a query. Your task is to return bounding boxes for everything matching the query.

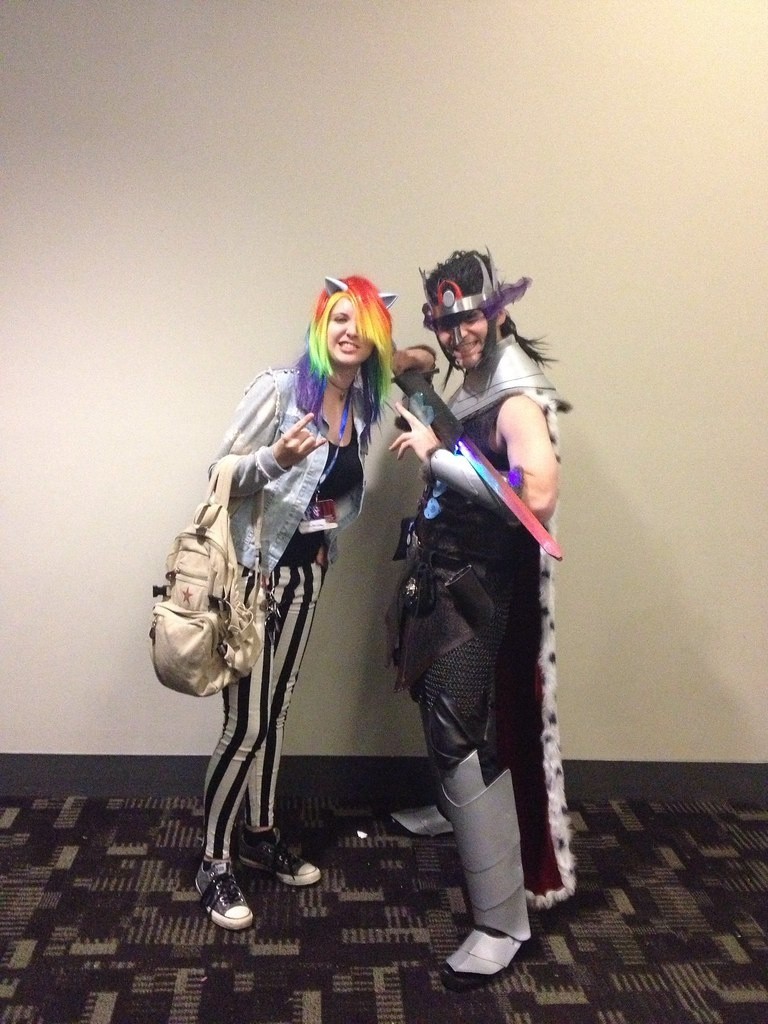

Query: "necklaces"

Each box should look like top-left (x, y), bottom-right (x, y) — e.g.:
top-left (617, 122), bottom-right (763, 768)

top-left (328, 376), bottom-right (355, 400)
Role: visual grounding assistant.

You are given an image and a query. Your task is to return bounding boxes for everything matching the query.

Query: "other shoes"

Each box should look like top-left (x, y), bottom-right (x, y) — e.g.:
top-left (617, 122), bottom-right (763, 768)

top-left (437, 925), bottom-right (523, 992)
top-left (389, 805), bottom-right (454, 838)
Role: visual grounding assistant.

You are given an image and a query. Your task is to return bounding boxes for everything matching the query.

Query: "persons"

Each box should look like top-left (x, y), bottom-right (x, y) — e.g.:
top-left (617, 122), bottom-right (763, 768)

top-left (196, 278), bottom-right (394, 929)
top-left (388, 249), bottom-right (564, 987)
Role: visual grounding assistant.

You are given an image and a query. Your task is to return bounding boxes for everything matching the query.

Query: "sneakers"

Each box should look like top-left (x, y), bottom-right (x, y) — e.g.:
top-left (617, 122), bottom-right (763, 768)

top-left (195, 858), bottom-right (252, 931)
top-left (239, 823), bottom-right (322, 886)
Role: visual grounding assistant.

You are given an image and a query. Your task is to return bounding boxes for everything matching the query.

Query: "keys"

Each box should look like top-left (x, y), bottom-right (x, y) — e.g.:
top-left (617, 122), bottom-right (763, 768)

top-left (264, 601), bottom-right (282, 639)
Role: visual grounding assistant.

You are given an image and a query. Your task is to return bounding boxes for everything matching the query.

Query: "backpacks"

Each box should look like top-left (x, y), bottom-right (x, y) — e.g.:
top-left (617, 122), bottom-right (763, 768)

top-left (149, 455), bottom-right (270, 698)
